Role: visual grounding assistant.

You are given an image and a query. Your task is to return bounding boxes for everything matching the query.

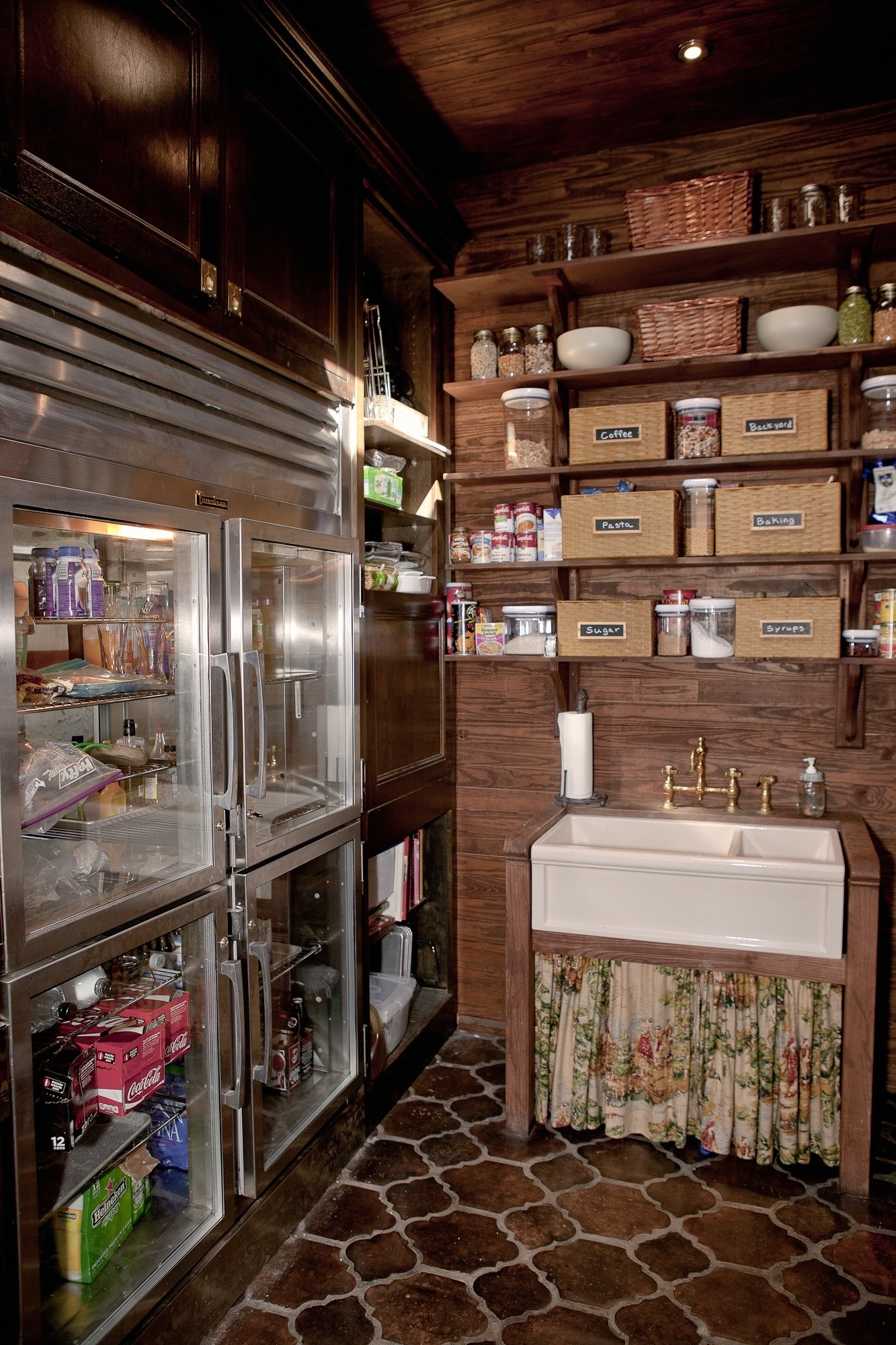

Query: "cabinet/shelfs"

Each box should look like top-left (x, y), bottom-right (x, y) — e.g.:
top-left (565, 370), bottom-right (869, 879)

top-left (0, 0), bottom-right (896, 750)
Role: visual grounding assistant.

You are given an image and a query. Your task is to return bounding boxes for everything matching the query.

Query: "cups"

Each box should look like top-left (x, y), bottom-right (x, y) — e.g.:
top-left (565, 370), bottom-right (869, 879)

top-left (96, 580), bottom-right (175, 686)
top-left (51, 1192), bottom-right (84, 1281)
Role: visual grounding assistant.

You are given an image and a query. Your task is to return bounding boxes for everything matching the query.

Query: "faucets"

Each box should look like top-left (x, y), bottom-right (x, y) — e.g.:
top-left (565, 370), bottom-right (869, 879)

top-left (660, 734), bottom-right (742, 814)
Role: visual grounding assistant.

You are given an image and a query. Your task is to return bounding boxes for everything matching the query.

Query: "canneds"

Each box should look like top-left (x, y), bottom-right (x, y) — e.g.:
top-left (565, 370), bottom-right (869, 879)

top-left (675, 397), bottom-right (722, 458)
top-left (450, 502), bottom-right (543, 564)
top-left (842, 629), bottom-right (882, 657)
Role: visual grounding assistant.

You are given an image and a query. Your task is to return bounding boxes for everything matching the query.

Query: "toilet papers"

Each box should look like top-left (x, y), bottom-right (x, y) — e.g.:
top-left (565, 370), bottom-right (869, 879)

top-left (556, 711), bottom-right (596, 800)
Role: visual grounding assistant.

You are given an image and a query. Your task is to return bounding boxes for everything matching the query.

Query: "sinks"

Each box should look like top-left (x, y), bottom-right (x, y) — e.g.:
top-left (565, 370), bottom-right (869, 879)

top-left (527, 812), bottom-right (740, 948)
top-left (729, 823), bottom-right (846, 959)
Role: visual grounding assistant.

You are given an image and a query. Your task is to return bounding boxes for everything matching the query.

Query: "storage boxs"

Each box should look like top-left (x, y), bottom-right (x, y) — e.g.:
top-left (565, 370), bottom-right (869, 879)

top-left (46, 1141), bottom-right (161, 1284)
top-left (543, 509), bottom-right (563, 561)
top-left (369, 971), bottom-right (417, 1056)
top-left (32, 979), bottom-right (188, 1153)
top-left (364, 465), bottom-right (403, 506)
top-left (261, 1028), bottom-right (314, 1096)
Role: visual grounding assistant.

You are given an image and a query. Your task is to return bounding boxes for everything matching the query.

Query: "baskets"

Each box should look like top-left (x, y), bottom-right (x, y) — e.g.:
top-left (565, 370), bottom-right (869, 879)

top-left (636, 294), bottom-right (746, 364)
top-left (735, 596), bottom-right (842, 659)
top-left (557, 600), bottom-right (661, 657)
top-left (569, 401), bottom-right (674, 466)
top-left (620, 169), bottom-right (761, 251)
top-left (721, 389), bottom-right (830, 457)
top-left (561, 490), bottom-right (683, 561)
top-left (716, 482), bottom-right (842, 556)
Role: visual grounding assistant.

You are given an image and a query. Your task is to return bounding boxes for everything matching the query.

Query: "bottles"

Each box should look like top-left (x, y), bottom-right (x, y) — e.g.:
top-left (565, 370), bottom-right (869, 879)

top-left (470, 323), bottom-right (554, 382)
top-left (142, 1074), bottom-right (188, 1171)
top-left (654, 589), bottom-right (736, 658)
top-left (674, 396), bottom-right (721, 459)
top-left (270, 992), bottom-right (284, 1018)
top-left (27, 545), bottom-right (106, 621)
top-left (59, 965), bottom-right (119, 1011)
top-left (290, 997), bottom-right (314, 1082)
top-left (838, 282), bottom-right (896, 345)
top-left (100, 943), bottom-right (166, 982)
top-left (681, 478), bottom-right (718, 556)
top-left (30, 986), bottom-right (78, 1034)
top-left (251, 597), bottom-right (266, 684)
top-left (500, 386), bottom-right (552, 470)
top-left (527, 223), bottom-right (608, 266)
top-left (268, 745), bottom-right (279, 785)
top-left (762, 183), bottom-right (861, 234)
top-left (130, 1175), bottom-right (152, 1226)
top-left (858, 374), bottom-right (896, 449)
top-left (64, 719), bottom-right (178, 821)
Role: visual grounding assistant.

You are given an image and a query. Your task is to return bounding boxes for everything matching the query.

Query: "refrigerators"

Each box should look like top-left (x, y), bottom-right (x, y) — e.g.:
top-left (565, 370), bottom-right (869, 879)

top-left (0, 241), bottom-right (361, 1345)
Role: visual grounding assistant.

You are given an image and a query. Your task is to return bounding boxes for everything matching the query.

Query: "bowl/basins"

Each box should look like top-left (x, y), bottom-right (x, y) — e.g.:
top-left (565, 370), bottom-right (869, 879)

top-left (557, 326), bottom-right (631, 370)
top-left (478, 640), bottom-right (503, 656)
top-left (756, 304), bottom-right (838, 351)
top-left (856, 525), bottom-right (896, 552)
top-left (363, 540), bottom-right (437, 595)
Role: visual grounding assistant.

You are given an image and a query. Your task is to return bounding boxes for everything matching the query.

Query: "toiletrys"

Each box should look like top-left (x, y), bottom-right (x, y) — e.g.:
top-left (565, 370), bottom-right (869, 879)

top-left (795, 757), bottom-right (826, 818)
